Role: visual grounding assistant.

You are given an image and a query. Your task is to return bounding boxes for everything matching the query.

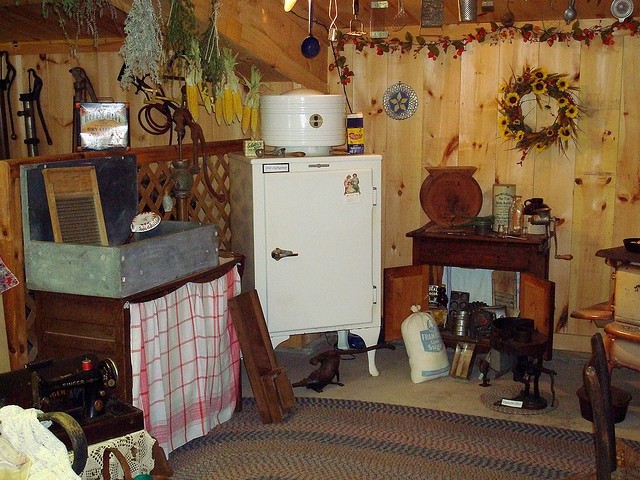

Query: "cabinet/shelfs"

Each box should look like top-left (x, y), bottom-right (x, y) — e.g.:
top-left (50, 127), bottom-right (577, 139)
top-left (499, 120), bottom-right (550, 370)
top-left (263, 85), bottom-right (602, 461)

top-left (383, 220), bottom-right (556, 361)
top-left (27, 257), bottom-right (244, 424)
top-left (226, 152), bottom-right (384, 377)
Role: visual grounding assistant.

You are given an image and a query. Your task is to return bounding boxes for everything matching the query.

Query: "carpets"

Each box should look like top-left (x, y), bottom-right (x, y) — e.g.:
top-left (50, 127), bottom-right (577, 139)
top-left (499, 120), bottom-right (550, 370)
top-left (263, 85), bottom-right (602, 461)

top-left (164, 397), bottom-right (635, 480)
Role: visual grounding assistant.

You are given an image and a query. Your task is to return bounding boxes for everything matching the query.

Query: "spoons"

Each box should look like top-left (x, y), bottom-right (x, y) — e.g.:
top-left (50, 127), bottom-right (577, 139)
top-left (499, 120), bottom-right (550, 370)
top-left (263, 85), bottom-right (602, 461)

top-left (256, 149), bottom-right (306, 158)
top-left (300, 0), bottom-right (320, 60)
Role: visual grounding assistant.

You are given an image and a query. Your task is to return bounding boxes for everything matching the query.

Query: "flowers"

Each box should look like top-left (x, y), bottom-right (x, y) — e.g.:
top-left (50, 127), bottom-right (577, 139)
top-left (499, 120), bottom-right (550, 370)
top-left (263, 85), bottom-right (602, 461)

top-left (492, 57), bottom-right (587, 169)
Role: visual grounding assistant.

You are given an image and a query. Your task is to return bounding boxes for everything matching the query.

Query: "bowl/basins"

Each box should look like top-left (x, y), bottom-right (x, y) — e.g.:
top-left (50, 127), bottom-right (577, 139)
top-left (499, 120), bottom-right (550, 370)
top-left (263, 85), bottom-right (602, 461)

top-left (574, 386), bottom-right (632, 424)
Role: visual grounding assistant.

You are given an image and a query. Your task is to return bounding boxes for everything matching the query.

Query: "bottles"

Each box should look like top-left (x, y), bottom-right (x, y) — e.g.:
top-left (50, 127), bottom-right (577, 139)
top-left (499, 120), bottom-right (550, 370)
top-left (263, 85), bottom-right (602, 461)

top-left (474, 217), bottom-right (489, 234)
top-left (509, 195), bottom-right (523, 234)
top-left (491, 183), bottom-right (515, 232)
top-left (346, 112), bottom-right (365, 154)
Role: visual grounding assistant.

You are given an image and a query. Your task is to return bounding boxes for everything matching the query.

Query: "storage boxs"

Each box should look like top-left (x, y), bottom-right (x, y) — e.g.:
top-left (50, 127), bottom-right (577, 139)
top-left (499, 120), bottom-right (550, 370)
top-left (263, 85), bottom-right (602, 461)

top-left (613, 264), bottom-right (640, 325)
